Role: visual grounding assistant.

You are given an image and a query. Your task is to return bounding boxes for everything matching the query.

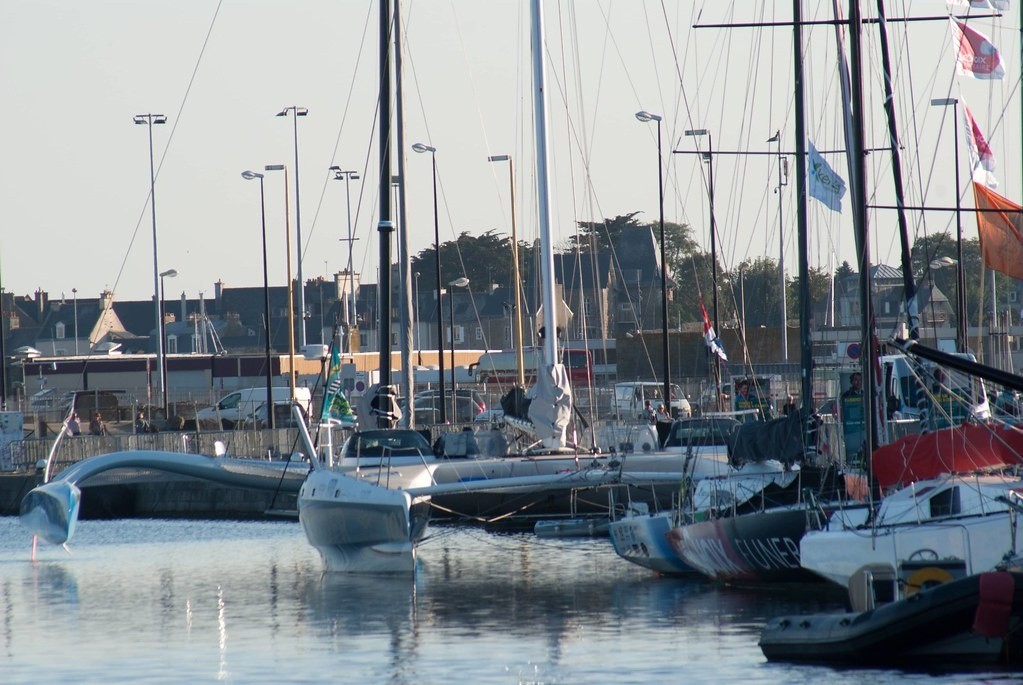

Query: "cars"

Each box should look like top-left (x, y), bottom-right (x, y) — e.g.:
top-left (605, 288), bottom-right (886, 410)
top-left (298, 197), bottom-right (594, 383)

top-left (338, 429), bottom-right (437, 470)
top-left (664, 417), bottom-right (745, 447)
top-left (401, 387), bottom-right (505, 428)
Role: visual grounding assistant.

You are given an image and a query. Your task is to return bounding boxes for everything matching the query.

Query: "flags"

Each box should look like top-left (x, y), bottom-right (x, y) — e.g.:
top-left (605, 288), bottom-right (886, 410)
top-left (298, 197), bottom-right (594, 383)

top-left (944, 0), bottom-right (1010, 12)
top-left (61, 393), bottom-right (77, 437)
top-left (961, 94), bottom-right (996, 191)
top-left (321, 343), bottom-right (354, 423)
top-left (808, 140), bottom-right (846, 214)
top-left (949, 14), bottom-right (1005, 79)
top-left (699, 298), bottom-right (728, 361)
top-left (973, 182), bottom-right (1023, 279)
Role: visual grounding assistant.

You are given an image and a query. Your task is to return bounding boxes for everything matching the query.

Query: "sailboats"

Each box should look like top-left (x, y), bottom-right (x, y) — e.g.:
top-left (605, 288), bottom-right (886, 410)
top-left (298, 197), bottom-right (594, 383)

top-left (533, 0), bottom-right (1023, 674)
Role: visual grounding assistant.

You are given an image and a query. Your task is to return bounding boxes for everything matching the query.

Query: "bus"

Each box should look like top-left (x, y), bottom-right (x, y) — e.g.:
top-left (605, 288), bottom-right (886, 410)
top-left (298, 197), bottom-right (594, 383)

top-left (469, 344), bottom-right (595, 387)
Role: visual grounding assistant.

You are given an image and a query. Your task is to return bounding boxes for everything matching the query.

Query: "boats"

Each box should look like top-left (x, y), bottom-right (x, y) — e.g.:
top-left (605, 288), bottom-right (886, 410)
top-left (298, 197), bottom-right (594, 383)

top-left (291, 407), bottom-right (431, 575)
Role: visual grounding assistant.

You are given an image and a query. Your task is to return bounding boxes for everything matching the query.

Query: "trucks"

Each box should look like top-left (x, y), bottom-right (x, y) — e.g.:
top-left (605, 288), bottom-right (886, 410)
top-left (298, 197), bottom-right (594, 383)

top-left (193, 387), bottom-right (313, 428)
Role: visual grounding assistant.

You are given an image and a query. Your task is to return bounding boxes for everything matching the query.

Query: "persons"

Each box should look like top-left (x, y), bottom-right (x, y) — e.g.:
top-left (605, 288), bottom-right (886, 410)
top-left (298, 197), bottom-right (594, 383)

top-left (89, 413), bottom-right (105, 436)
top-left (135, 411), bottom-right (150, 433)
top-left (842, 372), bottom-right (863, 396)
top-left (929, 368), bottom-right (948, 391)
top-left (636, 388), bottom-right (676, 423)
top-left (71, 411), bottom-right (81, 435)
top-left (783, 395), bottom-right (796, 416)
top-left (987, 387), bottom-right (1023, 417)
top-left (735, 380), bottom-right (756, 401)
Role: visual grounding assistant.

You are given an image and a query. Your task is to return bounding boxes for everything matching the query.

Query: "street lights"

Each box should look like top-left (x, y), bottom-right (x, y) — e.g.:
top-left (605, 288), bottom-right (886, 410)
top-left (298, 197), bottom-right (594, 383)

top-left (72, 288), bottom-right (78, 356)
top-left (159, 269), bottom-right (178, 421)
top-left (328, 166), bottom-right (360, 330)
top-left (487, 154), bottom-right (526, 390)
top-left (132, 113), bottom-right (164, 410)
top-left (415, 272), bottom-right (421, 367)
top-left (413, 143), bottom-right (446, 424)
top-left (636, 112), bottom-right (672, 419)
top-left (242, 105), bottom-right (311, 456)
top-left (684, 129), bottom-right (722, 411)
top-left (449, 278), bottom-right (470, 424)
top-left (929, 97), bottom-right (967, 352)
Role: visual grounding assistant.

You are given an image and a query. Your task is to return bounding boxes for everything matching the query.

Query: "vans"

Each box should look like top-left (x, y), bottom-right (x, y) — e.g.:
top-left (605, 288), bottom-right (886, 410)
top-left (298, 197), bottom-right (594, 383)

top-left (613, 382), bottom-right (692, 420)
